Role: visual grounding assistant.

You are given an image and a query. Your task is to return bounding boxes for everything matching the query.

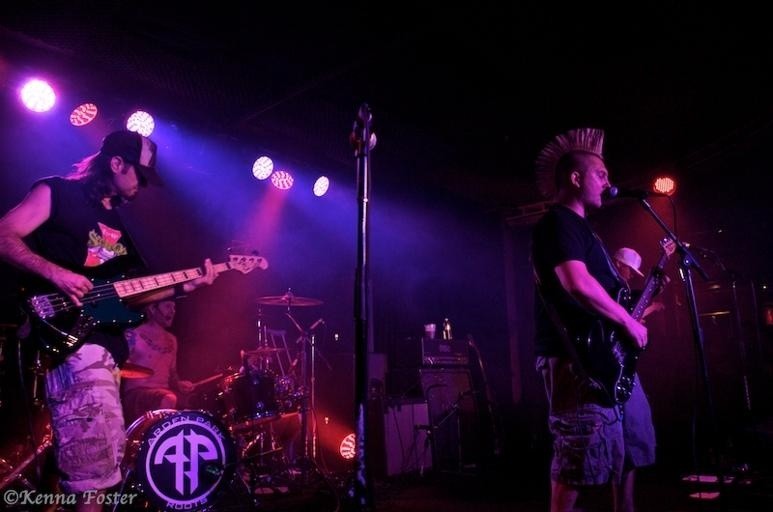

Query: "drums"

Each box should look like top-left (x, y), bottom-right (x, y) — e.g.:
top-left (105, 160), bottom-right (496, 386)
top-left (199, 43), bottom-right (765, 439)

top-left (1, 397), bottom-right (64, 511)
top-left (213, 364), bottom-right (280, 433)
top-left (122, 409), bottom-right (232, 510)
top-left (274, 376), bottom-right (301, 418)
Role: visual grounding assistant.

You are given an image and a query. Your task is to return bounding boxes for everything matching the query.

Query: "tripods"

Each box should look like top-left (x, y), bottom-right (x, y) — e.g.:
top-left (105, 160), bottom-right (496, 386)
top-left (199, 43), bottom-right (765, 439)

top-left (260, 314), bottom-right (332, 485)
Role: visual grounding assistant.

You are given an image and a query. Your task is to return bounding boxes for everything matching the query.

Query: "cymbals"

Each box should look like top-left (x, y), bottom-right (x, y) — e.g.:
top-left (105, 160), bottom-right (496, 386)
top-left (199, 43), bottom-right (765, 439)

top-left (241, 347), bottom-right (287, 354)
top-left (121, 362), bottom-right (153, 378)
top-left (259, 295), bottom-right (323, 308)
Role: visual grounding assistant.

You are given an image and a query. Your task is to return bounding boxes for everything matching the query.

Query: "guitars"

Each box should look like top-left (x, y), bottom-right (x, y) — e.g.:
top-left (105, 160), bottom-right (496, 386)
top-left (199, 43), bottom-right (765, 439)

top-left (17, 244), bottom-right (269, 357)
top-left (568, 234), bottom-right (678, 406)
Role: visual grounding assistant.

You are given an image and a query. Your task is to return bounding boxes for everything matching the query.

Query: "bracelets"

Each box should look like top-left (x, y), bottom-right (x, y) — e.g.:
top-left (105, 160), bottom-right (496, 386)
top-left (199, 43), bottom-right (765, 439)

top-left (175, 284), bottom-right (188, 300)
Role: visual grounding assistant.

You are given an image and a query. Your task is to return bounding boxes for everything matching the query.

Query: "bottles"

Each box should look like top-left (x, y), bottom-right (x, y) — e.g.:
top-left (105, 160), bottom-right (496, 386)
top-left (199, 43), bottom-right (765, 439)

top-left (342, 458), bottom-right (366, 511)
top-left (442, 318), bottom-right (454, 341)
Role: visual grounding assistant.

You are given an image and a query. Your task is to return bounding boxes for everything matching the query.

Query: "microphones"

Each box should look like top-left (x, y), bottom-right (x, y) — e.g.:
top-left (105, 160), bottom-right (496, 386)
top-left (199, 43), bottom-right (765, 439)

top-left (345, 101), bottom-right (377, 150)
top-left (416, 424), bottom-right (434, 431)
top-left (609, 186), bottom-right (651, 198)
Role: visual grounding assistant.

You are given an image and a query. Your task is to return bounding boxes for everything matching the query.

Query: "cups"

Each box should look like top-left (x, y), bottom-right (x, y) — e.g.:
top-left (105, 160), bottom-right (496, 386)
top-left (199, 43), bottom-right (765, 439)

top-left (424, 323), bottom-right (435, 338)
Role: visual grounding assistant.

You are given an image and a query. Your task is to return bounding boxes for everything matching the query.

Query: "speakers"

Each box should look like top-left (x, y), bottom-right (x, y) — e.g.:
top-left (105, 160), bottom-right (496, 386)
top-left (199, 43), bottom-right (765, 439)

top-left (371, 399), bottom-right (435, 477)
top-left (395, 368), bottom-right (480, 418)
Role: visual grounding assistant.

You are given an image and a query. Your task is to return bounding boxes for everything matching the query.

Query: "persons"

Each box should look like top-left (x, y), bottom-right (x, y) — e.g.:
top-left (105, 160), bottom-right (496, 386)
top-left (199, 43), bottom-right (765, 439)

top-left (531, 129), bottom-right (657, 509)
top-left (120, 297), bottom-right (197, 421)
top-left (0, 129), bottom-right (219, 512)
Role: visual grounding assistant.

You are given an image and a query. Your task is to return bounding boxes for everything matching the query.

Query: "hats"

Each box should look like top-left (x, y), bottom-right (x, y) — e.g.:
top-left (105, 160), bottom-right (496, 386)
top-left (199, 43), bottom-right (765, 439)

top-left (611, 247), bottom-right (645, 279)
top-left (98, 131), bottom-right (165, 188)
